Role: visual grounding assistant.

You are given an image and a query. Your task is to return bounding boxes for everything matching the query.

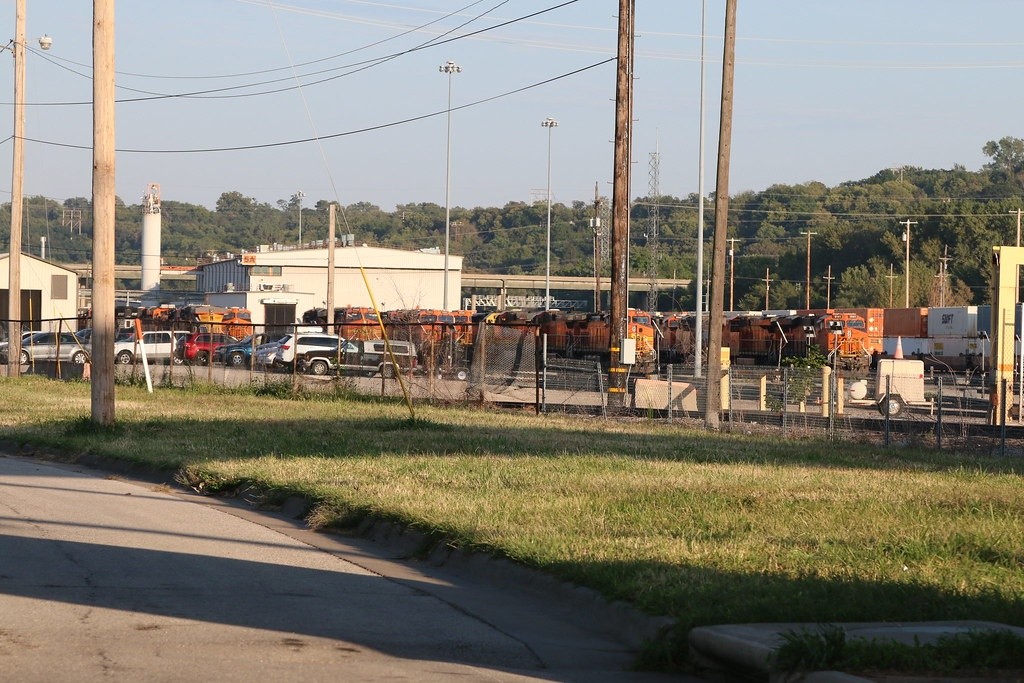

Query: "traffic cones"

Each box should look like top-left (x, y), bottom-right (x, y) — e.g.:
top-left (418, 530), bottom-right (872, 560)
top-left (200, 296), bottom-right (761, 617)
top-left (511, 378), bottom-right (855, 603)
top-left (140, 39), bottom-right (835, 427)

top-left (894, 335), bottom-right (904, 360)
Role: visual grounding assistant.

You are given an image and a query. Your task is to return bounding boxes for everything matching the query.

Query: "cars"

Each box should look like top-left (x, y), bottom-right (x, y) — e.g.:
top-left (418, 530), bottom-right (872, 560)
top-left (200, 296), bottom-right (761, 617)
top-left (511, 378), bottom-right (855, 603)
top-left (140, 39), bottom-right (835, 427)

top-left (275, 333), bottom-right (376, 377)
top-left (76, 329), bottom-right (92, 339)
top-left (20, 332), bottom-right (92, 365)
top-left (114, 331), bottom-right (190, 365)
top-left (255, 341), bottom-right (287, 369)
top-left (173, 333), bottom-right (238, 366)
top-left (0, 331), bottom-right (43, 364)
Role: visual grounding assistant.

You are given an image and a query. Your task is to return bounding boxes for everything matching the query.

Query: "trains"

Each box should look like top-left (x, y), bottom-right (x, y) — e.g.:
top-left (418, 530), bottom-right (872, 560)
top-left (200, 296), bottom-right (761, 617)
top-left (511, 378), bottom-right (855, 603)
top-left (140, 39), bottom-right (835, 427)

top-left (303, 306), bottom-right (868, 373)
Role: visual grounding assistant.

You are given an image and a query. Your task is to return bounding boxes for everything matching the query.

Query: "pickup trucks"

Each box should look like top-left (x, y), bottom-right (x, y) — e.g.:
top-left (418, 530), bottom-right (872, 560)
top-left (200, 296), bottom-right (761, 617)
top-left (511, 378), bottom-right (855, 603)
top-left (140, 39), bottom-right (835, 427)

top-left (215, 333), bottom-right (274, 368)
top-left (298, 340), bottom-right (418, 379)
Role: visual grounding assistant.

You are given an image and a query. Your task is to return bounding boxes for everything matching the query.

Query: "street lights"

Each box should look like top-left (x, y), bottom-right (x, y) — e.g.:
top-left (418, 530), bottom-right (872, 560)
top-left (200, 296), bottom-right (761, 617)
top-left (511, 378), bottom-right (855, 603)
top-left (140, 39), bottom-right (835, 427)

top-left (542, 118), bottom-right (559, 311)
top-left (439, 60), bottom-right (462, 310)
top-left (8, 33), bottom-right (53, 376)
